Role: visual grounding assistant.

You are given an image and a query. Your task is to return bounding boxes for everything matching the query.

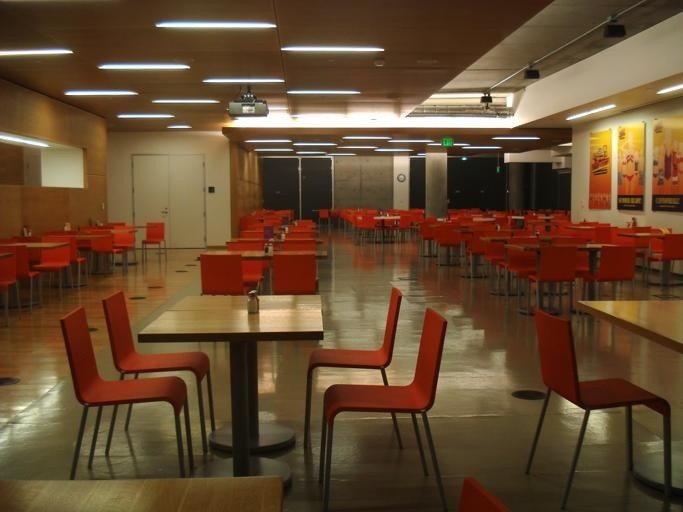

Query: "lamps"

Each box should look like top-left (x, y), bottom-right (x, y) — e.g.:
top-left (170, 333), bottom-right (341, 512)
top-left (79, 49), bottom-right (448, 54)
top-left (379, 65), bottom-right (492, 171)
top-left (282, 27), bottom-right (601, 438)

top-left (600, 19), bottom-right (627, 40)
top-left (522, 64), bottom-right (539, 80)
top-left (480, 92), bottom-right (492, 104)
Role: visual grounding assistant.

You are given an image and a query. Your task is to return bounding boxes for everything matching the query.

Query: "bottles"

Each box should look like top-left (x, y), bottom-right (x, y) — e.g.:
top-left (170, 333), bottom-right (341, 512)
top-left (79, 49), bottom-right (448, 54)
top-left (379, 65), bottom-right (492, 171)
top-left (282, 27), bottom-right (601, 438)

top-left (275, 228), bottom-right (286, 240)
top-left (70, 224), bottom-right (79, 233)
top-left (248, 289), bottom-right (260, 312)
top-left (22, 225), bottom-right (27, 237)
top-left (375, 207), bottom-right (390, 217)
top-left (263, 243), bottom-right (273, 255)
top-left (28, 229), bottom-right (32, 237)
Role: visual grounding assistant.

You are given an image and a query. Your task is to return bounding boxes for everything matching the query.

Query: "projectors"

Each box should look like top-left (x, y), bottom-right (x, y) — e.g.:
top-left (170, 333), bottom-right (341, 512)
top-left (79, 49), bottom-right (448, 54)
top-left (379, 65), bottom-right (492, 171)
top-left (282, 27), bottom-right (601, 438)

top-left (228, 100), bottom-right (269, 117)
